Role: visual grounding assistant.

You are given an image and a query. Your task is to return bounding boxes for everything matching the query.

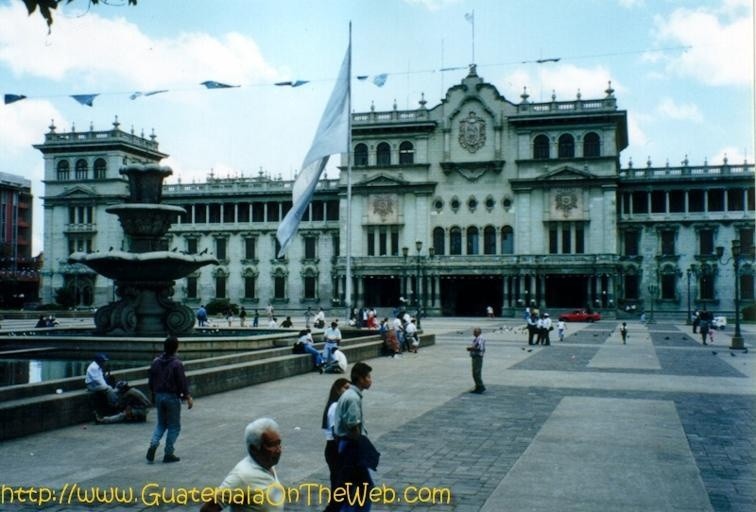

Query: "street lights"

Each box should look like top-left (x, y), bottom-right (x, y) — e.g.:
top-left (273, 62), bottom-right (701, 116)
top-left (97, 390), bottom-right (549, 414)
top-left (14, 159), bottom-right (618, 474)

top-left (687, 264), bottom-right (718, 324)
top-left (716, 240), bottom-right (756, 349)
top-left (402, 242), bottom-right (435, 330)
top-left (646, 285), bottom-right (657, 324)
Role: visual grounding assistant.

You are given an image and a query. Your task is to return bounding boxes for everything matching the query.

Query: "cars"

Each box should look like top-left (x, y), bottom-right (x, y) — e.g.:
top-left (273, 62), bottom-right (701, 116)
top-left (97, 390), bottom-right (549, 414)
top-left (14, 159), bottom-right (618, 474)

top-left (70, 303), bottom-right (110, 313)
top-left (559, 308), bottom-right (601, 323)
top-left (24, 302), bottom-right (41, 309)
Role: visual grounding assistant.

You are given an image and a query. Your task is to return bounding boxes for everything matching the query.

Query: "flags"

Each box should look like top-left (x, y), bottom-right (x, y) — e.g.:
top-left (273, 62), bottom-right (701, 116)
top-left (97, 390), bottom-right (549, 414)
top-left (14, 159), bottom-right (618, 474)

top-left (275, 47), bottom-right (353, 259)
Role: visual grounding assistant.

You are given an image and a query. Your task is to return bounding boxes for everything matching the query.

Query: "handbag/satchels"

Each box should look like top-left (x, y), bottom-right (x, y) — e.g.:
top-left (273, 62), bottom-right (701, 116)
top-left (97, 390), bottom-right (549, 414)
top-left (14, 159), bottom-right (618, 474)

top-left (293, 343), bottom-right (304, 354)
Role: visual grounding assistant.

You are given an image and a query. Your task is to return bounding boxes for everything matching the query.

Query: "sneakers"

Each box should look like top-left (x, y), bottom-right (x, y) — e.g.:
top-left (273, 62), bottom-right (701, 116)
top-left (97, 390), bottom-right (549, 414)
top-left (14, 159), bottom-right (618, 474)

top-left (92, 409), bottom-right (100, 424)
top-left (315, 361), bottom-right (342, 374)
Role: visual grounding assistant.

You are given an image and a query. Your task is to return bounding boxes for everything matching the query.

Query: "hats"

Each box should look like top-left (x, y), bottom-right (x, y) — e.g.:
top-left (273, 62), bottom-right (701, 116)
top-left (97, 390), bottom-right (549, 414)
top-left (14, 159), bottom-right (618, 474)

top-left (94, 351), bottom-right (109, 361)
top-left (113, 380), bottom-right (127, 392)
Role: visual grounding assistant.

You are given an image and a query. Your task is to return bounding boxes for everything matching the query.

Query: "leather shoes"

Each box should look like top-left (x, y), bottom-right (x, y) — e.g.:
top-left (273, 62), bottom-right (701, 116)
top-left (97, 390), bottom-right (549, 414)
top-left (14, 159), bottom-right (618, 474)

top-left (476, 386), bottom-right (485, 393)
top-left (163, 453), bottom-right (180, 461)
top-left (146, 447), bottom-right (156, 461)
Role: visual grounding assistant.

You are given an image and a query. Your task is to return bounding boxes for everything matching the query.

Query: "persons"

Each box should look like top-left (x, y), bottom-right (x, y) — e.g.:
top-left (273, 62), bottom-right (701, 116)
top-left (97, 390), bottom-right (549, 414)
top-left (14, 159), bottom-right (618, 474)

top-left (34, 315), bottom-right (58, 336)
top-left (321, 377), bottom-right (353, 512)
top-left (486, 305), bottom-right (496, 321)
top-left (334, 361), bottom-right (375, 512)
top-left (145, 336), bottom-right (193, 463)
top-left (466, 328), bottom-right (486, 394)
top-left (691, 305), bottom-right (717, 345)
top-left (199, 418), bottom-right (285, 512)
top-left (641, 312), bottom-right (650, 325)
top-left (196, 303), bottom-right (420, 375)
top-left (85, 353), bottom-right (119, 409)
top-left (95, 381), bottom-right (151, 424)
top-left (527, 308), bottom-right (566, 346)
top-left (621, 322), bottom-right (627, 345)
